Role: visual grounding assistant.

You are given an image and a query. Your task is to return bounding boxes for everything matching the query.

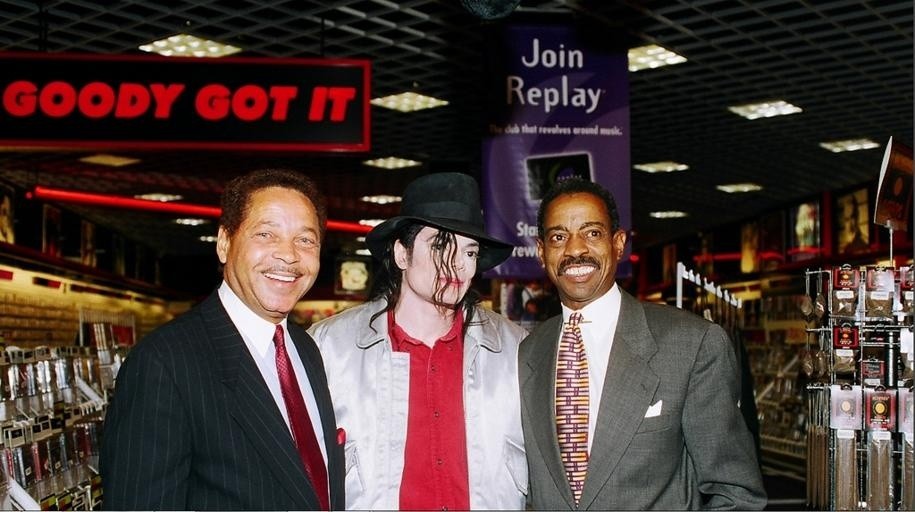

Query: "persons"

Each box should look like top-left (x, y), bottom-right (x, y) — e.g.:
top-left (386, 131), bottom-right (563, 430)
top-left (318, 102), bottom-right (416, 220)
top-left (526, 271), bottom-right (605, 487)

top-left (97, 167), bottom-right (346, 510)
top-left (516, 180), bottom-right (769, 511)
top-left (303, 172), bottom-right (530, 511)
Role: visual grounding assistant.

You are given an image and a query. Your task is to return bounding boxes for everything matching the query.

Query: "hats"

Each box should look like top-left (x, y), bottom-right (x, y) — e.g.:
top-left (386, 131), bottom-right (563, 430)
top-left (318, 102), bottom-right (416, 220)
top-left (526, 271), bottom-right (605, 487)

top-left (365, 173), bottom-right (514, 274)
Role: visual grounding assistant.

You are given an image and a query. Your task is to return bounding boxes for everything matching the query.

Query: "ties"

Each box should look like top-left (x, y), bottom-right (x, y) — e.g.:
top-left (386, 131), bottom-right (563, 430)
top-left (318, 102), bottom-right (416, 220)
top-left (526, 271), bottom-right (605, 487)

top-left (555, 312), bottom-right (590, 510)
top-left (272, 325), bottom-right (329, 509)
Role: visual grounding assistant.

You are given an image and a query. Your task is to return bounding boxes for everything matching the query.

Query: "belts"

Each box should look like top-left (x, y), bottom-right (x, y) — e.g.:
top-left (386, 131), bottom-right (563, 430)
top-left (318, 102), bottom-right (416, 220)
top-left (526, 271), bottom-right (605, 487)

top-left (807, 289), bottom-right (914, 510)
